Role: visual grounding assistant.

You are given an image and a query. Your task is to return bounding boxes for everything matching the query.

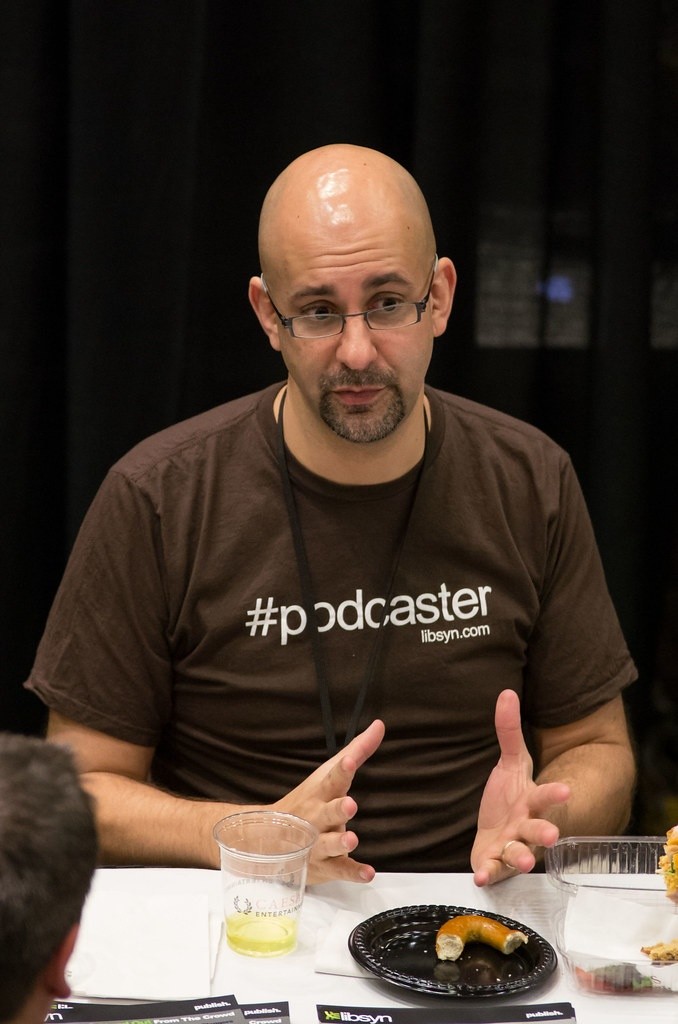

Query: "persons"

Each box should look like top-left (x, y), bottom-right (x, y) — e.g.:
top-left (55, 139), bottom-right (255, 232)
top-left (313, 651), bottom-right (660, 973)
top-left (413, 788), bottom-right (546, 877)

top-left (23, 145), bottom-right (637, 886)
top-left (0, 732), bottom-right (102, 1024)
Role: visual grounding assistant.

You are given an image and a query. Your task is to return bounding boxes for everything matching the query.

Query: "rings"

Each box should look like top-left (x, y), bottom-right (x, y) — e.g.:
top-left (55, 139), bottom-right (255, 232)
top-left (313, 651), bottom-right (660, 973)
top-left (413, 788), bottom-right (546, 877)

top-left (501, 840), bottom-right (517, 869)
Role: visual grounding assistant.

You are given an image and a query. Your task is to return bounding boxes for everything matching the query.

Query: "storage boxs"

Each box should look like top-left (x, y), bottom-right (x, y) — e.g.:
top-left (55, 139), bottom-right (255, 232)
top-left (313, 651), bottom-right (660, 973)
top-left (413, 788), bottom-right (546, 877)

top-left (543, 835), bottom-right (678, 998)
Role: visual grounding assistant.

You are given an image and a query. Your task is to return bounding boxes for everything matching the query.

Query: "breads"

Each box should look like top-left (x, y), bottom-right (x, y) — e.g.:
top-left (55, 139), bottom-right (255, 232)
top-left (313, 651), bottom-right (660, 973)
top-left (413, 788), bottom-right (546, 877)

top-left (641, 936), bottom-right (678, 966)
top-left (659, 824), bottom-right (678, 908)
top-left (435, 915), bottom-right (530, 961)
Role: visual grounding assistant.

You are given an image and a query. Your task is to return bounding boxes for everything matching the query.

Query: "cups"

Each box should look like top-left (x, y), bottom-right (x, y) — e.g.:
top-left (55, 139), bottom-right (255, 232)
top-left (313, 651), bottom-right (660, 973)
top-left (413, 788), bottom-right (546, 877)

top-left (212, 811), bottom-right (319, 959)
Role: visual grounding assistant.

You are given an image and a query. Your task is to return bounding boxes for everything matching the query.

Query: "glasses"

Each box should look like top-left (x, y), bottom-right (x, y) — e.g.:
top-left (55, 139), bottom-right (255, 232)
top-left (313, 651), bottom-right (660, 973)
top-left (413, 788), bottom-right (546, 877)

top-left (261, 255), bottom-right (439, 340)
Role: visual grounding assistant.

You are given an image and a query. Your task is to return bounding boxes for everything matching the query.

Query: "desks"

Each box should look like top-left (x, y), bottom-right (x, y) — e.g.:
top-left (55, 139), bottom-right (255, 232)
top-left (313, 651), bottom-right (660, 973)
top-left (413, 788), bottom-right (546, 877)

top-left (57, 867), bottom-right (678, 1024)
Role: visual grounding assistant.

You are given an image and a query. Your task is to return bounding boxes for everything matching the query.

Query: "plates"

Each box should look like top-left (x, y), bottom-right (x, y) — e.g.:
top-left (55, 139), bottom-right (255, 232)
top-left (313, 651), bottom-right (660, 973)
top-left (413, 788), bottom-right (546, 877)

top-left (347, 904), bottom-right (559, 999)
